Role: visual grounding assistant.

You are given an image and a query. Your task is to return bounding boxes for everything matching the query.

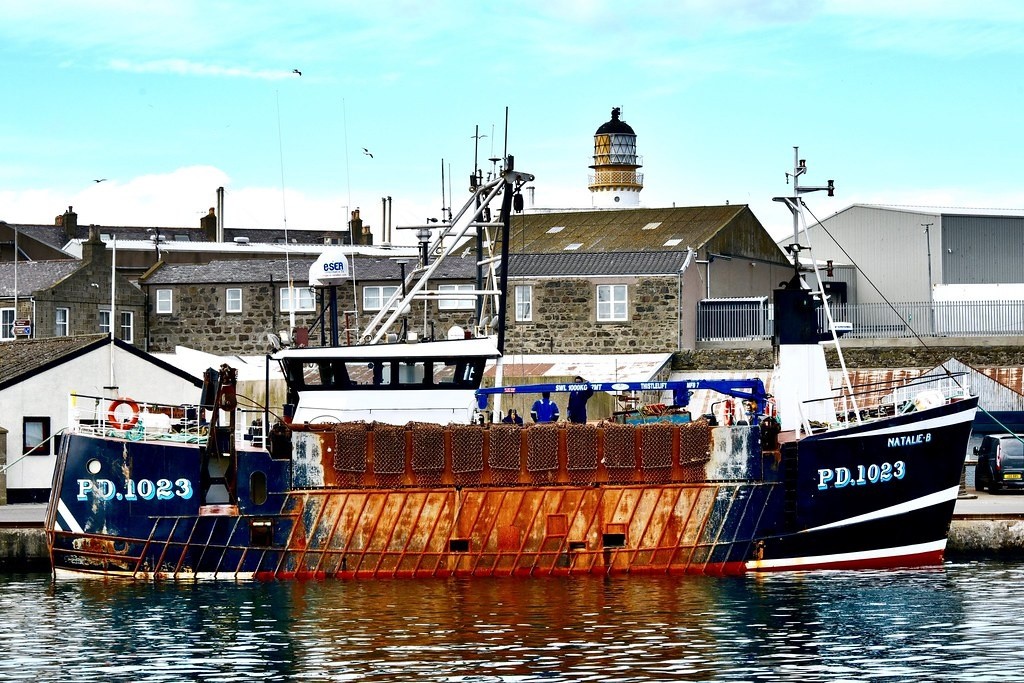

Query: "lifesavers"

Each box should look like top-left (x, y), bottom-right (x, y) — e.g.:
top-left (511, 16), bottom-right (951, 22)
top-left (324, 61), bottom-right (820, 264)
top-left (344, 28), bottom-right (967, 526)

top-left (723, 398), bottom-right (736, 426)
top-left (107, 396), bottom-right (140, 430)
top-left (766, 397), bottom-right (777, 418)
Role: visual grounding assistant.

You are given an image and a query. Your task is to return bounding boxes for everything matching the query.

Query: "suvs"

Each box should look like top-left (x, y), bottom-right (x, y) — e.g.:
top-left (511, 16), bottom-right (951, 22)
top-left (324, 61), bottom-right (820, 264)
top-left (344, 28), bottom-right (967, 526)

top-left (972, 433), bottom-right (1024, 495)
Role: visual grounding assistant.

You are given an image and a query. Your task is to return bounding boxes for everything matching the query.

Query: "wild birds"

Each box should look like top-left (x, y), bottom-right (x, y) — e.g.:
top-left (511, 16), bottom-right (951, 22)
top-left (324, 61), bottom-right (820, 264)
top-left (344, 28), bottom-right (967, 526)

top-left (91, 179), bottom-right (108, 183)
top-left (470, 135), bottom-right (488, 139)
top-left (292, 69), bottom-right (301, 76)
top-left (362, 147), bottom-right (373, 158)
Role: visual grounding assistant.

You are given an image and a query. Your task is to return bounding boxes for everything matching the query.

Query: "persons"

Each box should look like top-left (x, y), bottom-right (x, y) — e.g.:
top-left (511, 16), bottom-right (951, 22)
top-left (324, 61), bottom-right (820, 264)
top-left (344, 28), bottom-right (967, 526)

top-left (567, 376), bottom-right (593, 425)
top-left (376, 325), bottom-right (388, 343)
top-left (502, 409), bottom-right (523, 425)
top-left (531, 392), bottom-right (560, 424)
top-left (463, 326), bottom-right (476, 339)
top-left (479, 330), bottom-right (484, 337)
top-left (248, 419), bottom-right (270, 436)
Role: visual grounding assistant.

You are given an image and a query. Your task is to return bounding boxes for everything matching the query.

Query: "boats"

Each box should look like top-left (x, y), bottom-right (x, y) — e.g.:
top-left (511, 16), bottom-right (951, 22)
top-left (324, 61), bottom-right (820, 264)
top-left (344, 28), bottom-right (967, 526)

top-left (42, 106), bottom-right (981, 581)
